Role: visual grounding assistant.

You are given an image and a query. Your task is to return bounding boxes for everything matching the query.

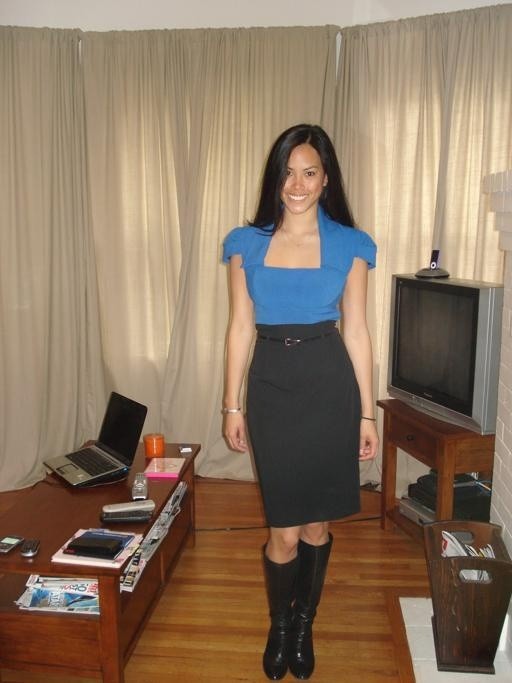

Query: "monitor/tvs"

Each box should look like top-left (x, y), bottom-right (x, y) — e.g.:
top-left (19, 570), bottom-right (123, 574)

top-left (387, 273), bottom-right (504, 436)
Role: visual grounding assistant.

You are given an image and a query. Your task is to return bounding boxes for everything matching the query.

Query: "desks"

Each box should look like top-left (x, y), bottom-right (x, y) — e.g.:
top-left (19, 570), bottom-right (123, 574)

top-left (0, 440), bottom-right (201, 683)
top-left (373, 395), bottom-right (495, 547)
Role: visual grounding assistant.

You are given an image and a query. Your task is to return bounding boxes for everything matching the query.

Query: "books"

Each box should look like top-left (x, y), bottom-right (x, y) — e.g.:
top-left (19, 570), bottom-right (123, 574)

top-left (51, 525), bottom-right (144, 570)
top-left (15, 575), bottom-right (100, 614)
top-left (119, 481), bottom-right (192, 592)
top-left (140, 455), bottom-right (188, 479)
top-left (442, 529), bottom-right (501, 586)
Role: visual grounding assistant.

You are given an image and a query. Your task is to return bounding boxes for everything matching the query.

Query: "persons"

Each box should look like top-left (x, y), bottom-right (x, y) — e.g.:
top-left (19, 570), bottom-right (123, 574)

top-left (218, 121), bottom-right (381, 681)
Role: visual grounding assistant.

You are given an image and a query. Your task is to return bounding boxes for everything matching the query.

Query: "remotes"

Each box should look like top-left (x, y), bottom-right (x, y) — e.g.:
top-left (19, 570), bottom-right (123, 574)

top-left (102, 499), bottom-right (155, 513)
top-left (100, 511), bottom-right (152, 523)
top-left (132, 479), bottom-right (148, 500)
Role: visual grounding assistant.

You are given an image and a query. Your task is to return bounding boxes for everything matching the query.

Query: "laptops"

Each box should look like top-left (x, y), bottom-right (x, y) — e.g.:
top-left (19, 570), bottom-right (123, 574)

top-left (42, 391), bottom-right (147, 487)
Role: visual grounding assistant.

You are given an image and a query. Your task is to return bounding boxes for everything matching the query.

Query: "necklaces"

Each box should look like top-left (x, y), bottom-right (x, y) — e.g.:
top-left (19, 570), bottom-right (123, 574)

top-left (277, 222), bottom-right (319, 250)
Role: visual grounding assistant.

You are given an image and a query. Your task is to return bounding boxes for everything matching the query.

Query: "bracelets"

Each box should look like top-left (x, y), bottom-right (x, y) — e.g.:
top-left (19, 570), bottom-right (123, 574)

top-left (360, 412), bottom-right (378, 424)
top-left (218, 405), bottom-right (243, 418)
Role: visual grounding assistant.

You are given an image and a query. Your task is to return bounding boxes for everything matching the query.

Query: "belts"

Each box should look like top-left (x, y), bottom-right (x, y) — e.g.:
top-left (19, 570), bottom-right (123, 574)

top-left (259, 333), bottom-right (334, 346)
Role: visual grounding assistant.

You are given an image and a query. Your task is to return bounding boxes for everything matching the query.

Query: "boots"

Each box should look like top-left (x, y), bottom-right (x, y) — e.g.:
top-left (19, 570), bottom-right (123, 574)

top-left (263, 543), bottom-right (300, 680)
top-left (289, 531), bottom-right (333, 680)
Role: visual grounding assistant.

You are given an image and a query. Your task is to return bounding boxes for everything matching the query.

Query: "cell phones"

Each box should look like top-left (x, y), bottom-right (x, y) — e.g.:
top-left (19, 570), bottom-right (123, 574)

top-left (179, 444), bottom-right (192, 453)
top-left (21, 538), bottom-right (40, 557)
top-left (0, 536), bottom-right (24, 553)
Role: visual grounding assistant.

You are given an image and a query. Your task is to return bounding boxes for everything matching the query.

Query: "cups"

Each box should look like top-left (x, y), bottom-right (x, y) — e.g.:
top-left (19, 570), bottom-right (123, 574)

top-left (143, 432), bottom-right (165, 459)
